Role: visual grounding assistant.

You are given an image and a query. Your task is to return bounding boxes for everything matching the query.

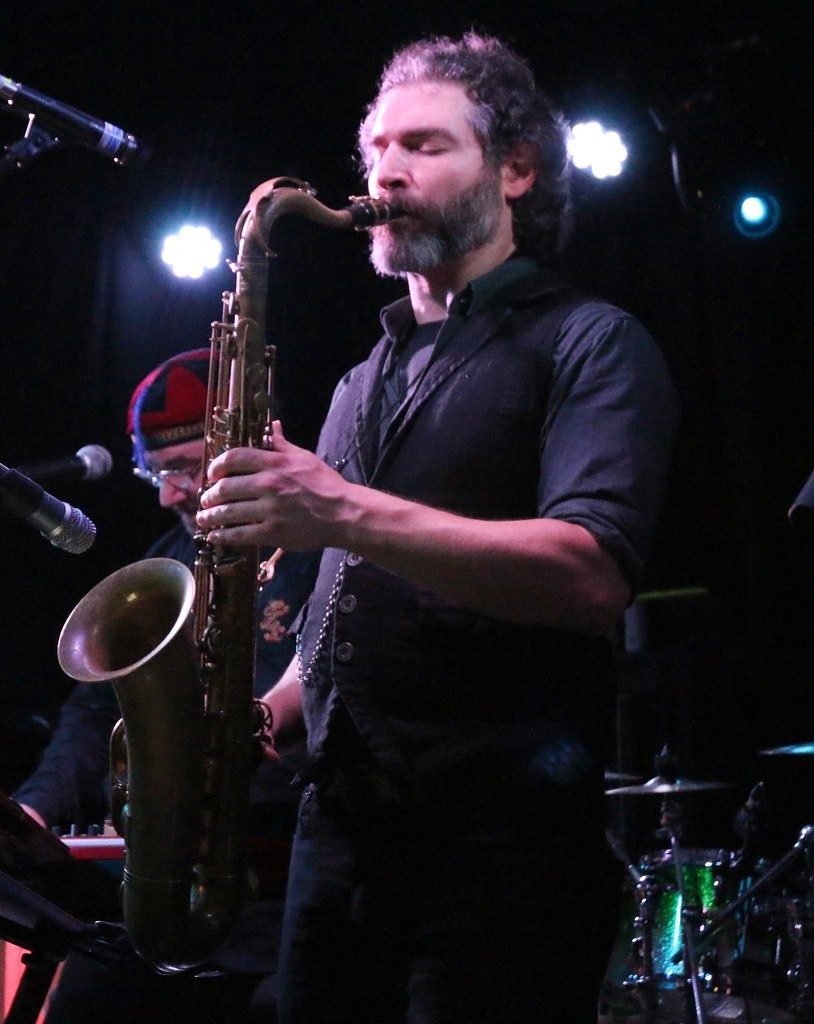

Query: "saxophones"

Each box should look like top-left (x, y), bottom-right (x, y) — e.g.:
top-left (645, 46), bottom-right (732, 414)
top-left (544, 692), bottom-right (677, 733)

top-left (56, 174), bottom-right (403, 971)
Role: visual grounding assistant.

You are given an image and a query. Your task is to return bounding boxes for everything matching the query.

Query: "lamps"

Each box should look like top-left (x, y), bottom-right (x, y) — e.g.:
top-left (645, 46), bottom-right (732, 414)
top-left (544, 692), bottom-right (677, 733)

top-left (563, 119), bottom-right (629, 181)
top-left (162, 225), bottom-right (224, 282)
top-left (733, 191), bottom-right (782, 240)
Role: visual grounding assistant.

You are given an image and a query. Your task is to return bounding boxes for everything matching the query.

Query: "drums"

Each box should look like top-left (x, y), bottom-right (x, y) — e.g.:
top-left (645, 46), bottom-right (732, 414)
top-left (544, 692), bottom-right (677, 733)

top-left (637, 845), bottom-right (779, 1004)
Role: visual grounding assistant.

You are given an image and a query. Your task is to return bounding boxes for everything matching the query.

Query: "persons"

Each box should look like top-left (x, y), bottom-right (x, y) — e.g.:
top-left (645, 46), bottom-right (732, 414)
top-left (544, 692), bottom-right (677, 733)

top-left (7, 347), bottom-right (310, 839)
top-left (195, 27), bottom-right (680, 1023)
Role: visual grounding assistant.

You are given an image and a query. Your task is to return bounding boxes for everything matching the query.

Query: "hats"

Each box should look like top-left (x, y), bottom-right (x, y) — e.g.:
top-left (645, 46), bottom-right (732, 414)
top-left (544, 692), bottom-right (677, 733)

top-left (128, 347), bottom-right (232, 451)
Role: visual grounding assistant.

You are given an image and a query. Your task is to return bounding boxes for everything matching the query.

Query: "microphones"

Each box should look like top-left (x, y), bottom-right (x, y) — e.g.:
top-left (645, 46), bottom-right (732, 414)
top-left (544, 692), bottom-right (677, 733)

top-left (12, 443), bottom-right (114, 486)
top-left (0, 462), bottom-right (97, 554)
top-left (0, 74), bottom-right (140, 169)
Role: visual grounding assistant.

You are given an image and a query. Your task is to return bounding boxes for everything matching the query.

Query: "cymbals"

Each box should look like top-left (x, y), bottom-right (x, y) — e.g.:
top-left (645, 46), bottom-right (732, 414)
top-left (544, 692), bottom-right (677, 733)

top-left (760, 744), bottom-right (814, 755)
top-left (603, 775), bottom-right (718, 793)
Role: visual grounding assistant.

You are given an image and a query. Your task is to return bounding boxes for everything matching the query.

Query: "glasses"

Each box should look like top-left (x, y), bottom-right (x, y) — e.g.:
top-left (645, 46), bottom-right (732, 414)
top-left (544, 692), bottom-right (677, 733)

top-left (133, 460), bottom-right (203, 487)
